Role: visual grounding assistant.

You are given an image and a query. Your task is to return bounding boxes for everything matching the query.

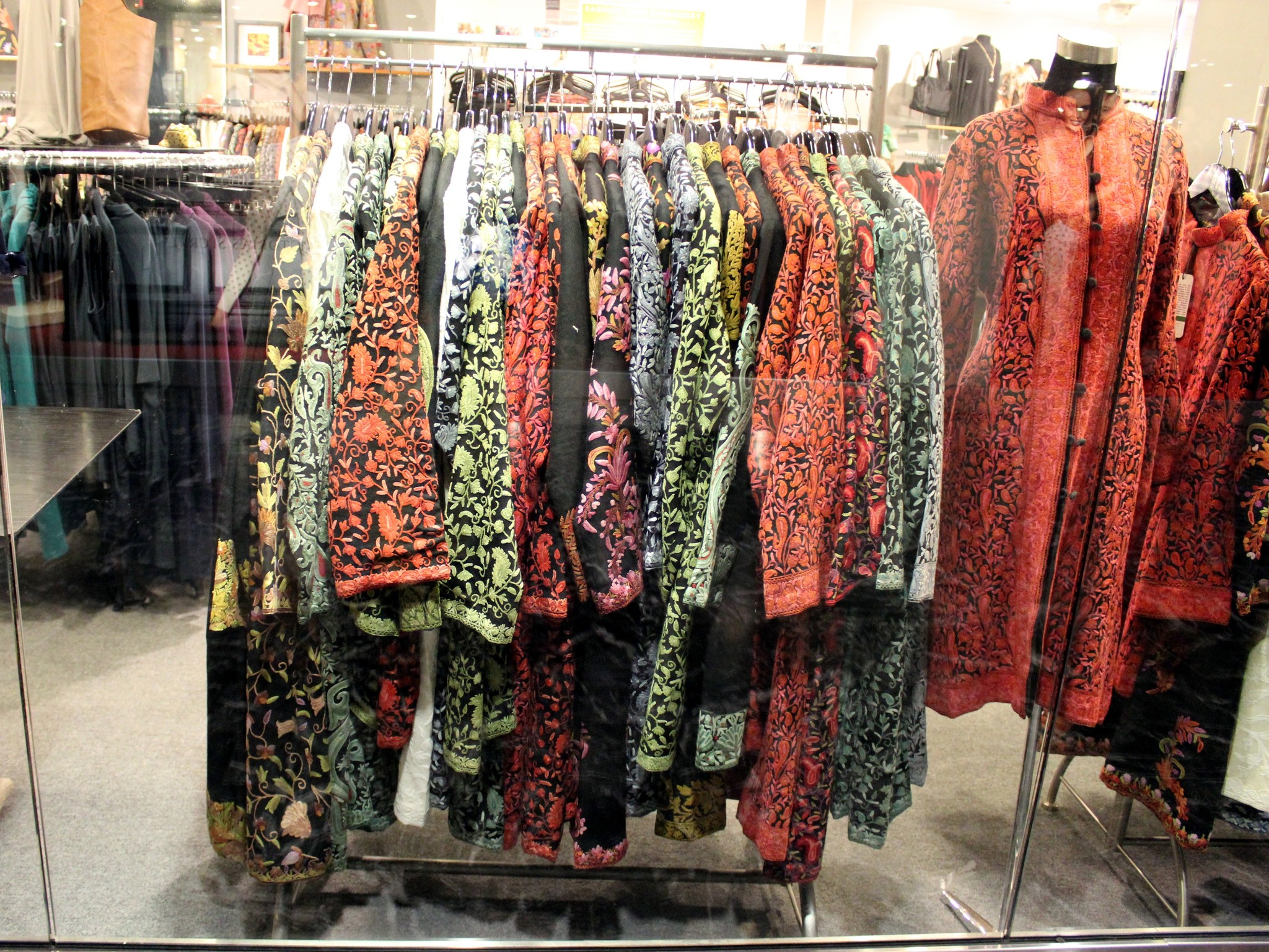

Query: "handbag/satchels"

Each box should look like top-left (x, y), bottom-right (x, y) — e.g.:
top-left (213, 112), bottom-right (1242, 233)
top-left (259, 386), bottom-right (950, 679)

top-left (908, 51), bottom-right (952, 118)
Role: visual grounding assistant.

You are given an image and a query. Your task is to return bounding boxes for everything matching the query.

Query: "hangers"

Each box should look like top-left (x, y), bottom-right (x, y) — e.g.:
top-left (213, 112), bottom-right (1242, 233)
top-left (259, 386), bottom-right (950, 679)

top-left (0, 99), bottom-right (291, 283)
top-left (1187, 120), bottom-right (1251, 217)
top-left (304, 56), bottom-right (947, 178)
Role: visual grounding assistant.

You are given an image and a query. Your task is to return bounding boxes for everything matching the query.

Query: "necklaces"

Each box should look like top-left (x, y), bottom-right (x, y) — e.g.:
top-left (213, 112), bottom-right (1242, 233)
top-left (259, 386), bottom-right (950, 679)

top-left (975, 39), bottom-right (997, 82)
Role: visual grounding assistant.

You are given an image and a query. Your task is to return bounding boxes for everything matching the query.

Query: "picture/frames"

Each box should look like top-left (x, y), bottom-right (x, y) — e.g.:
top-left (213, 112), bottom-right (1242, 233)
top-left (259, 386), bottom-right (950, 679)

top-left (234, 19), bottom-right (283, 66)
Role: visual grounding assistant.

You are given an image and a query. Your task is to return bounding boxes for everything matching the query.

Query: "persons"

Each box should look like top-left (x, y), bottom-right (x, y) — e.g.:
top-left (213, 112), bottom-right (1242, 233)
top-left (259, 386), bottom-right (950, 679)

top-left (283, 0), bottom-right (381, 71)
top-left (1003, 58), bottom-right (1048, 107)
top-left (924, 34), bottom-right (1191, 728)
top-left (945, 35), bottom-right (1001, 141)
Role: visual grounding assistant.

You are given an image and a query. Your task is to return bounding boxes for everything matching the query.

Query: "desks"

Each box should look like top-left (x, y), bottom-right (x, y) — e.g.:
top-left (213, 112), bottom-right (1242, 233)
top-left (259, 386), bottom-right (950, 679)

top-left (0, 407), bottom-right (141, 548)
